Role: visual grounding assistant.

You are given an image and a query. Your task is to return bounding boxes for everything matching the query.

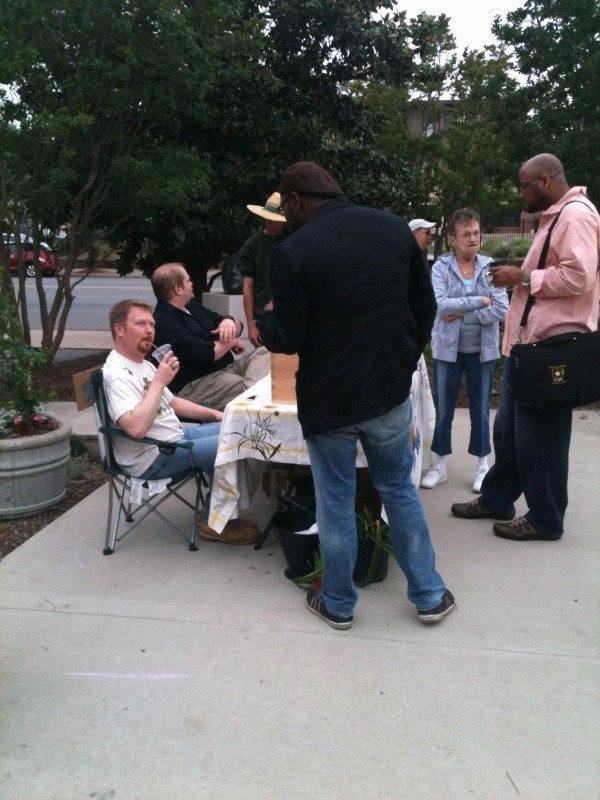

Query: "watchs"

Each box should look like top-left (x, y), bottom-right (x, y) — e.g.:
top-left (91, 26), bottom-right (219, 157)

top-left (521, 270), bottom-right (531, 286)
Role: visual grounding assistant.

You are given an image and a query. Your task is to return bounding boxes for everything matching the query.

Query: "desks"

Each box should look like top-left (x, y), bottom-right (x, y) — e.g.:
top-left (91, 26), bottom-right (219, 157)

top-left (205, 350), bottom-right (438, 556)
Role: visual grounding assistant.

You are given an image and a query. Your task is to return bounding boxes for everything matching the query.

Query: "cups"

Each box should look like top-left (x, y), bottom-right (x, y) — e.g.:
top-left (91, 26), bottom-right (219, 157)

top-left (151, 344), bottom-right (180, 373)
top-left (490, 259), bottom-right (510, 279)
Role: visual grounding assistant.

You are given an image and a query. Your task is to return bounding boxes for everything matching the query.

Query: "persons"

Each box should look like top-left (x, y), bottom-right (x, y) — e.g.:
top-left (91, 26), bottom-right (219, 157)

top-left (408, 219), bottom-right (436, 251)
top-left (448, 152), bottom-right (600, 541)
top-left (255, 161), bottom-right (455, 631)
top-left (102, 298), bottom-right (262, 544)
top-left (420, 207), bottom-right (508, 492)
top-left (236, 191), bottom-right (288, 346)
top-left (151, 261), bottom-right (271, 412)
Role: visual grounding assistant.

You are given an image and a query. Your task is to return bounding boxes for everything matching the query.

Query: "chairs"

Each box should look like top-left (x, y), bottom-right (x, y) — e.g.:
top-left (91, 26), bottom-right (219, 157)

top-left (92, 363), bottom-right (211, 555)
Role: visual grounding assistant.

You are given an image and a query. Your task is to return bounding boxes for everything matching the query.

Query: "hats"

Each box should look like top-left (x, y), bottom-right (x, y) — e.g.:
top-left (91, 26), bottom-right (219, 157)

top-left (408, 219), bottom-right (437, 231)
top-left (246, 192), bottom-right (288, 222)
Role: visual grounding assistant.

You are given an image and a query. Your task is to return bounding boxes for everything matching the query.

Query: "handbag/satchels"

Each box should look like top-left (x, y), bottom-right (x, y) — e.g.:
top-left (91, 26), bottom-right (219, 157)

top-left (504, 330), bottom-right (600, 408)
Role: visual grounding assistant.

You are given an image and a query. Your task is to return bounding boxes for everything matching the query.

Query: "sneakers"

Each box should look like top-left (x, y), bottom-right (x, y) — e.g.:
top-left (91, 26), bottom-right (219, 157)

top-left (197, 511), bottom-right (260, 544)
top-left (472, 468), bottom-right (490, 493)
top-left (418, 588), bottom-right (456, 623)
top-left (420, 464), bottom-right (447, 488)
top-left (492, 515), bottom-right (563, 541)
top-left (451, 498), bottom-right (516, 520)
top-left (305, 589), bottom-right (353, 630)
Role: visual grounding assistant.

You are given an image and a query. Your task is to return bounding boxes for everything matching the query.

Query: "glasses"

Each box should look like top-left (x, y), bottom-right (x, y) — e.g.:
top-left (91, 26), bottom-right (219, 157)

top-left (276, 197), bottom-right (294, 215)
top-left (454, 231), bottom-right (481, 238)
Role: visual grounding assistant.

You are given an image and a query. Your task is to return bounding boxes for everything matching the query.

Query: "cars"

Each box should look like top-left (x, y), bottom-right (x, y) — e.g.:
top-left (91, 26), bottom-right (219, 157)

top-left (2, 243), bottom-right (57, 277)
